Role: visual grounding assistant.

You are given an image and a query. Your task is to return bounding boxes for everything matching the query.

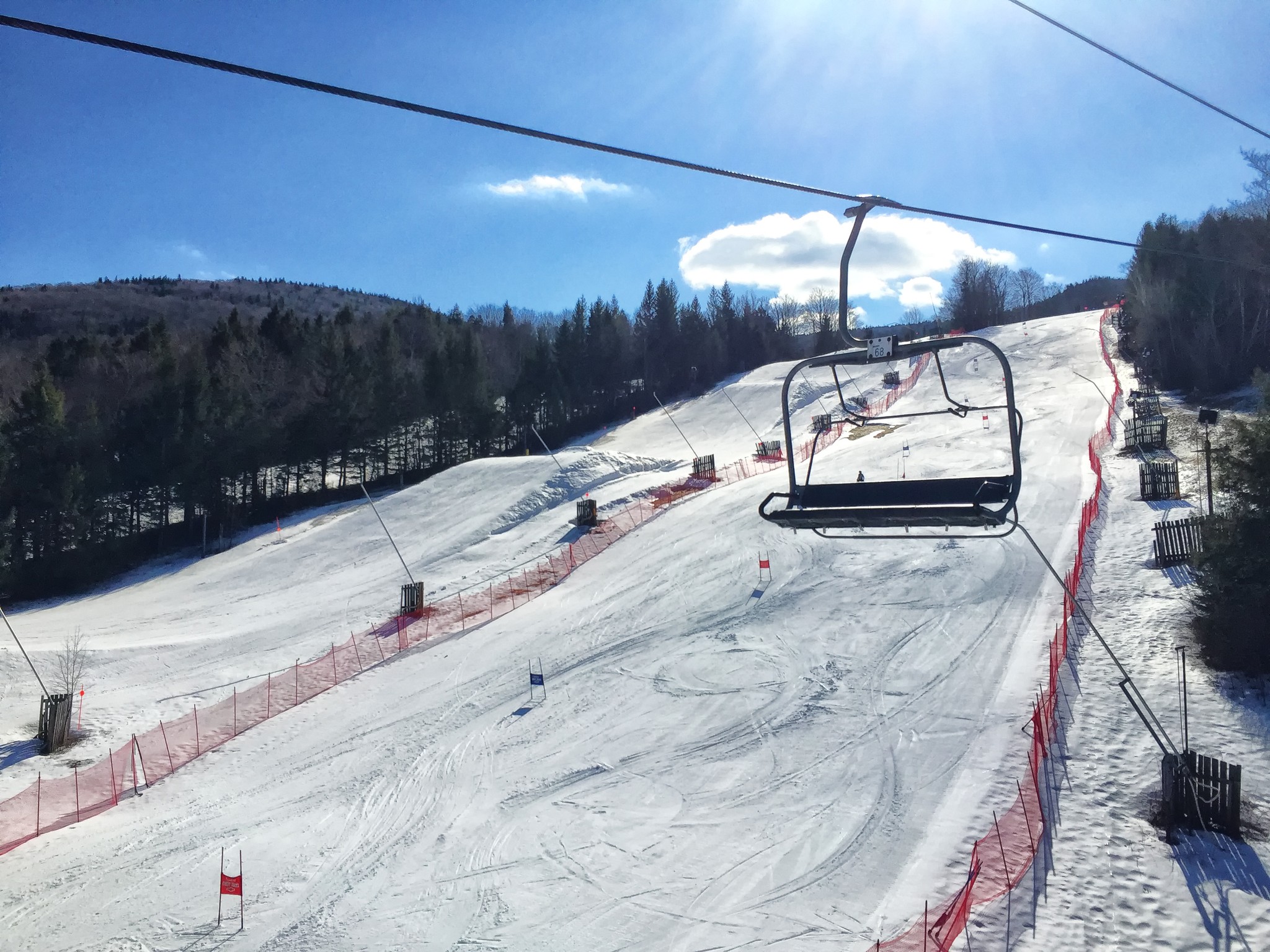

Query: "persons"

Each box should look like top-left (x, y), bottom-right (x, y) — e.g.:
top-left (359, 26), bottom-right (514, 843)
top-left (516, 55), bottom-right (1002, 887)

top-left (856, 470), bottom-right (864, 482)
